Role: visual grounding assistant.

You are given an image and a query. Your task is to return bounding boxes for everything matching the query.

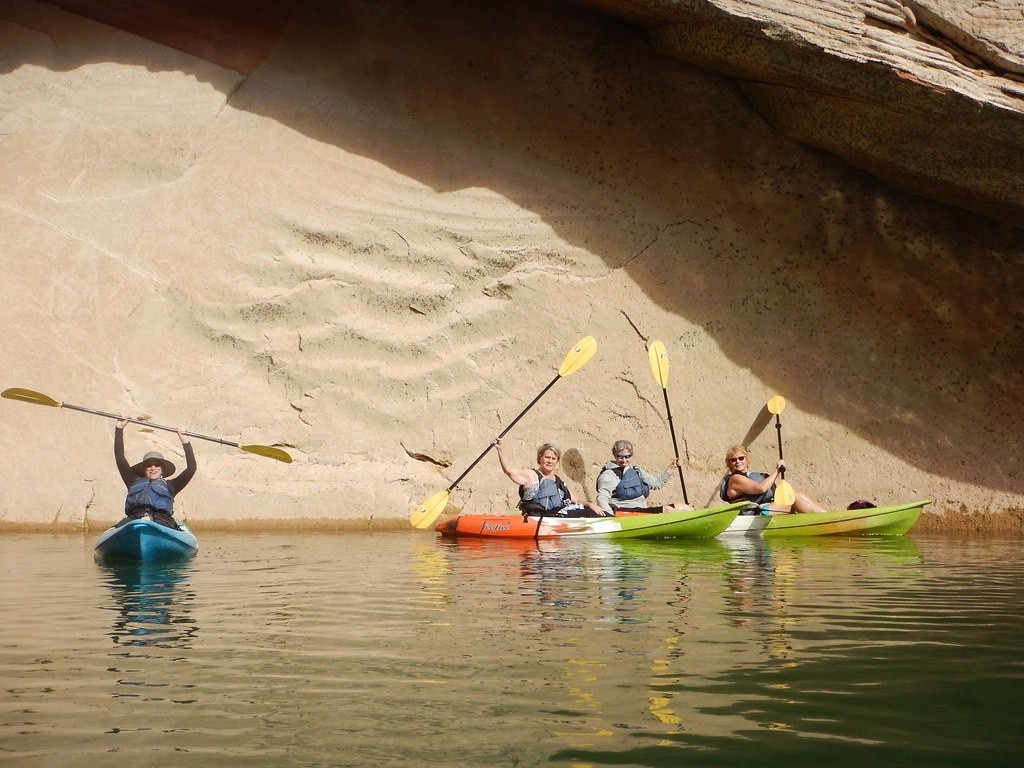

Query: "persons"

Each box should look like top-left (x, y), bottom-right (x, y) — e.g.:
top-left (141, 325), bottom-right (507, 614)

top-left (720, 445), bottom-right (826, 515)
top-left (596, 440), bottom-right (694, 516)
top-left (490, 437), bottom-right (615, 518)
top-left (113, 414), bottom-right (197, 531)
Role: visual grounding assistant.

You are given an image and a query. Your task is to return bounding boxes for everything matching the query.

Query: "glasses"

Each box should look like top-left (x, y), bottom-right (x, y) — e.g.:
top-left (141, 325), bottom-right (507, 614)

top-left (615, 454), bottom-right (630, 459)
top-left (144, 463), bottom-right (162, 468)
top-left (729, 456), bottom-right (745, 462)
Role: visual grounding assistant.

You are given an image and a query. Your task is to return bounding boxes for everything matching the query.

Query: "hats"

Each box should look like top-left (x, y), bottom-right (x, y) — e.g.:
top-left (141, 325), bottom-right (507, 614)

top-left (131, 451), bottom-right (176, 478)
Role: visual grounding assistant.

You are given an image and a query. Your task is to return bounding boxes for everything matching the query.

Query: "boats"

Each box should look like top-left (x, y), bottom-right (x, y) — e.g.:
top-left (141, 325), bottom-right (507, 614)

top-left (432, 498), bottom-right (760, 538)
top-left (718, 498), bottom-right (934, 541)
top-left (92, 517), bottom-right (203, 564)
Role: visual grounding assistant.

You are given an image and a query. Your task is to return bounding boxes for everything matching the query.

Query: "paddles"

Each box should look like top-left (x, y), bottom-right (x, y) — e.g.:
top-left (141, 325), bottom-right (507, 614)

top-left (767, 396), bottom-right (798, 507)
top-left (408, 335), bottom-right (597, 531)
top-left (1, 387), bottom-right (293, 465)
top-left (649, 339), bottom-right (691, 506)
